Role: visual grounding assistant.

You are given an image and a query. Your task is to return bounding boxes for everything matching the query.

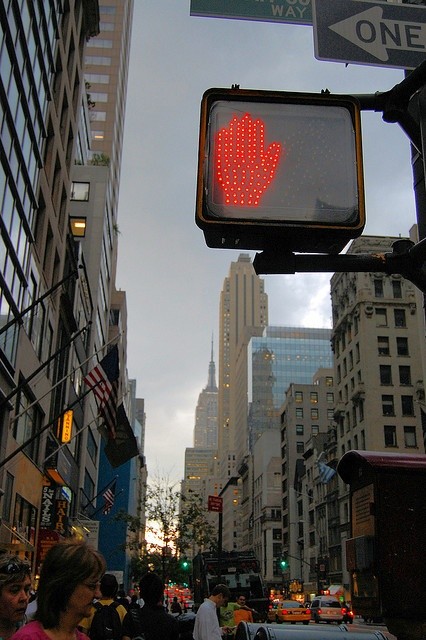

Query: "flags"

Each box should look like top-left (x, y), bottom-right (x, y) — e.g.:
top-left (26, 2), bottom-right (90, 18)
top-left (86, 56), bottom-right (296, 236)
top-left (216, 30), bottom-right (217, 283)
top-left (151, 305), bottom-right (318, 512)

top-left (102, 479), bottom-right (116, 514)
top-left (82, 343), bottom-right (119, 443)
top-left (98, 402), bottom-right (139, 468)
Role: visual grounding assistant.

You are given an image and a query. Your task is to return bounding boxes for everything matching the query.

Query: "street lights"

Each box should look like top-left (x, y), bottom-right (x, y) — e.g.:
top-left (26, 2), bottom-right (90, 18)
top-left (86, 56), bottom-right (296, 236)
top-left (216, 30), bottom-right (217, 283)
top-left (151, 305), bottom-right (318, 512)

top-left (281, 551), bottom-right (316, 573)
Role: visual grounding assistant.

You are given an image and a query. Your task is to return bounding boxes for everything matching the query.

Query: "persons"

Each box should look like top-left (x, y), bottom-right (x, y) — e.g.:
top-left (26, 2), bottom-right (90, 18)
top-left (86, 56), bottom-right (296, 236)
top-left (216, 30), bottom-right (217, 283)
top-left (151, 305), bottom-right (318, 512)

top-left (192, 584), bottom-right (231, 640)
top-left (234, 595), bottom-right (254, 625)
top-left (219, 598), bottom-right (254, 639)
top-left (123, 574), bottom-right (176, 640)
top-left (119, 587), bottom-right (139, 607)
top-left (165, 596), bottom-right (189, 615)
top-left (0, 555), bottom-right (28, 639)
top-left (12, 544), bottom-right (107, 640)
top-left (77, 574), bottom-right (131, 640)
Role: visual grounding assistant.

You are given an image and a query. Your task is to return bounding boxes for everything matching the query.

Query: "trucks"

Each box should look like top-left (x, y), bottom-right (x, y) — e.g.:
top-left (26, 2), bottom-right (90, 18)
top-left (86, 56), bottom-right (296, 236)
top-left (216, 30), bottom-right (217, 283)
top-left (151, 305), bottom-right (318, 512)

top-left (192, 551), bottom-right (268, 623)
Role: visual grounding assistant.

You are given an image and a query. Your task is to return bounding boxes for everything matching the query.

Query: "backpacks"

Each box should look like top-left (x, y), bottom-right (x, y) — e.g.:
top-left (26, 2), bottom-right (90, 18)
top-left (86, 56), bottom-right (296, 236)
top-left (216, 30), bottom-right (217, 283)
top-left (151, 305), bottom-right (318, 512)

top-left (88, 601), bottom-right (122, 640)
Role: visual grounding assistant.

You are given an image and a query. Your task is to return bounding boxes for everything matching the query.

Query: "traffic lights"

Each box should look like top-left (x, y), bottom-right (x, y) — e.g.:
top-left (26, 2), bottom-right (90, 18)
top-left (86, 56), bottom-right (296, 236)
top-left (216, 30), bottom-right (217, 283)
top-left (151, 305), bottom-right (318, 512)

top-left (196, 87), bottom-right (366, 252)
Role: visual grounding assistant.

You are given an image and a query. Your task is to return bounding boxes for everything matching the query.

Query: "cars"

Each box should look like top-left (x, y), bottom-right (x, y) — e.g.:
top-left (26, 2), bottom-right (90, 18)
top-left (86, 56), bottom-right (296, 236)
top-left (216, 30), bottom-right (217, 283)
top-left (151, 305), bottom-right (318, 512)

top-left (266, 601), bottom-right (310, 624)
top-left (340, 604), bottom-right (353, 624)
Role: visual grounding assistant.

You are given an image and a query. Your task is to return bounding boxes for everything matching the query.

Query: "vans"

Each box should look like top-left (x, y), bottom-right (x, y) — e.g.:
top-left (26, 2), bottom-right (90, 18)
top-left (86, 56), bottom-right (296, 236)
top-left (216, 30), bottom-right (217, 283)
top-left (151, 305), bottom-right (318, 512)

top-left (307, 596), bottom-right (342, 624)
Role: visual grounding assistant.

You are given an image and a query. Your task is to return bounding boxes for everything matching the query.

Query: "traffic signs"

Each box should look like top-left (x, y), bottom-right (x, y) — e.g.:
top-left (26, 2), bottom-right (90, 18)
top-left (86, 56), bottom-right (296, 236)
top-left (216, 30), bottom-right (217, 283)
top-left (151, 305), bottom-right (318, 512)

top-left (207, 495), bottom-right (223, 512)
top-left (188, 1), bottom-right (313, 26)
top-left (309, 0), bottom-right (425, 71)
